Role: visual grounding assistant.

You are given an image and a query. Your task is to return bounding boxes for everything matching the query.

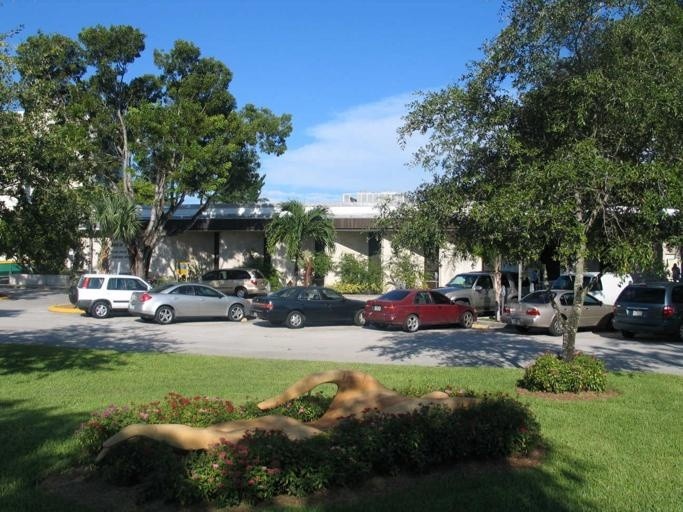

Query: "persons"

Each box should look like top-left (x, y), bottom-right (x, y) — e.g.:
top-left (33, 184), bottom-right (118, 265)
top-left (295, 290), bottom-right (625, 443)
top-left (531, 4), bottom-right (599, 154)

top-left (671, 262), bottom-right (680, 285)
top-left (531, 269), bottom-right (540, 285)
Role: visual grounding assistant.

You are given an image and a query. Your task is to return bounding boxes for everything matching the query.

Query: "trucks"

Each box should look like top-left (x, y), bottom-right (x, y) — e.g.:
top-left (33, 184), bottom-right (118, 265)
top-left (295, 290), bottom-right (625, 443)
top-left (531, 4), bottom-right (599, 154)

top-left (550, 272), bottom-right (633, 306)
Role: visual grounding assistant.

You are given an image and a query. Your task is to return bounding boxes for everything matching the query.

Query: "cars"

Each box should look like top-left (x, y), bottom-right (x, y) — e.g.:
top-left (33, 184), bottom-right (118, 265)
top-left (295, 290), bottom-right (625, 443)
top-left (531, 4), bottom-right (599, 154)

top-left (128, 283), bottom-right (253, 324)
top-left (500, 290), bottom-right (620, 336)
top-left (248, 286), bottom-right (369, 328)
top-left (361, 289), bottom-right (476, 332)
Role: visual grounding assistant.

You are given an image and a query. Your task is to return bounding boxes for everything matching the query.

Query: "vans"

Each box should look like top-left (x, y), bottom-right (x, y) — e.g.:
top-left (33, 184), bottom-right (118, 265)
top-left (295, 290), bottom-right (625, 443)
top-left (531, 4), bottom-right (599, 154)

top-left (430, 270), bottom-right (528, 313)
top-left (611, 282), bottom-right (682, 338)
top-left (193, 267), bottom-right (270, 299)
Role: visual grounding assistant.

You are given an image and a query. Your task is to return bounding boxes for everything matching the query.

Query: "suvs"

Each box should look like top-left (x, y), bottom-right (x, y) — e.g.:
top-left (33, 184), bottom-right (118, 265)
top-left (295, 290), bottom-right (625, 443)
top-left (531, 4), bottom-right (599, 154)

top-left (68, 273), bottom-right (153, 319)
